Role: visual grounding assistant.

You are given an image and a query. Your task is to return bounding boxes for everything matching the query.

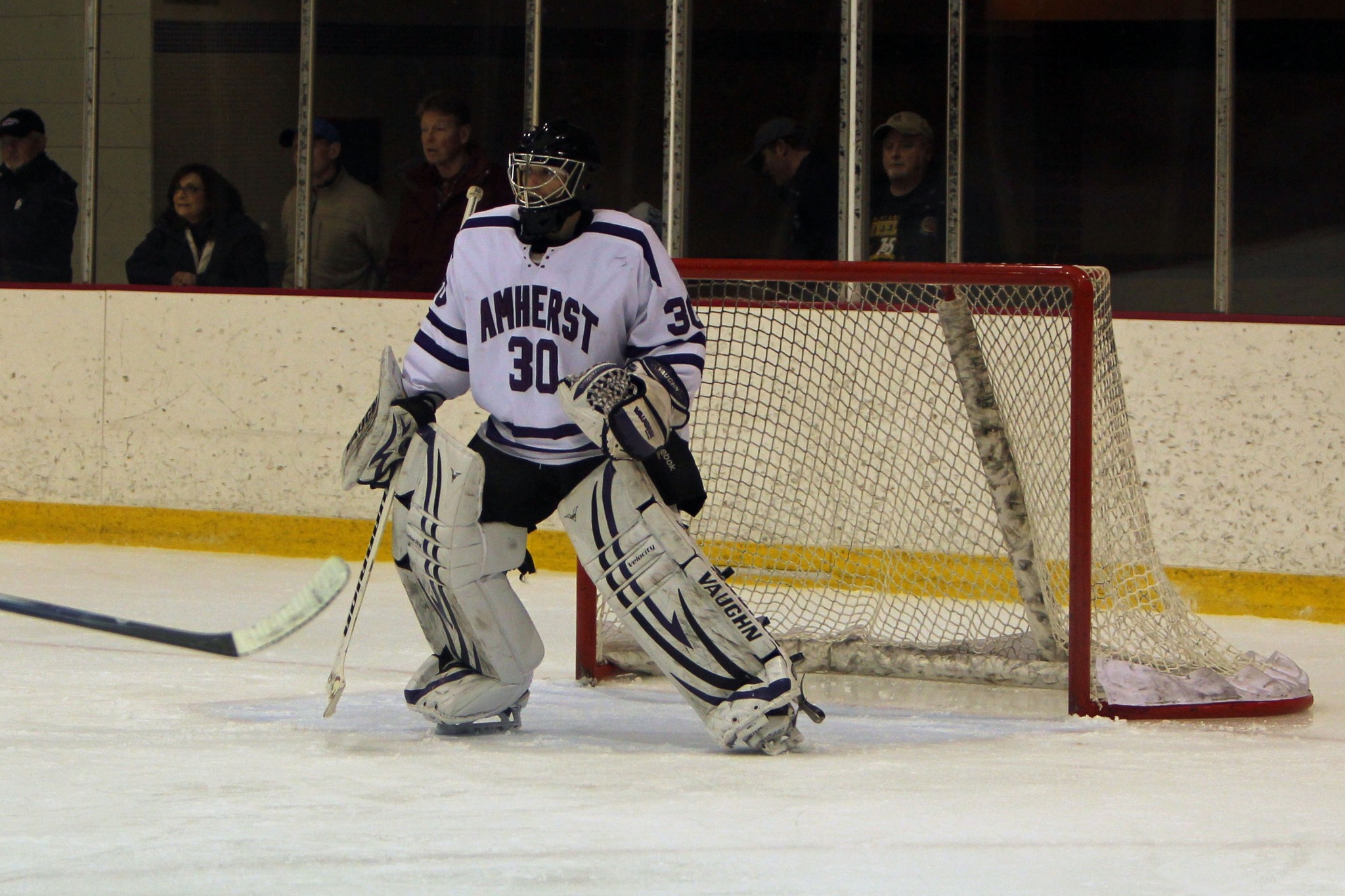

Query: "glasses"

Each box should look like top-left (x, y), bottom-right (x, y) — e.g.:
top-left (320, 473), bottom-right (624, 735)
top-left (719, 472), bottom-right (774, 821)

top-left (172, 184), bottom-right (206, 196)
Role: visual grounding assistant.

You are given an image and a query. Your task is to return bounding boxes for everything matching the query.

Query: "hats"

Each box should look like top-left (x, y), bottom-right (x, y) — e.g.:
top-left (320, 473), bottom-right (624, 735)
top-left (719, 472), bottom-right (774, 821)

top-left (746, 118), bottom-right (813, 167)
top-left (872, 111), bottom-right (934, 149)
top-left (0, 108), bottom-right (45, 139)
top-left (279, 118), bottom-right (343, 148)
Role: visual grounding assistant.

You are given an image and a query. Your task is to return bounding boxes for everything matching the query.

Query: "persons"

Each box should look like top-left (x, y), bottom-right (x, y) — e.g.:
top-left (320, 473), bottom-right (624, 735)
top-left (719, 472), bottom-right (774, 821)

top-left (339, 122), bottom-right (826, 755)
top-left (740, 117), bottom-right (839, 302)
top-left (814, 111), bottom-right (999, 304)
top-left (377, 88), bottom-right (502, 294)
top-left (125, 164), bottom-right (268, 288)
top-left (277, 114), bottom-right (396, 290)
top-left (0, 107), bottom-right (79, 283)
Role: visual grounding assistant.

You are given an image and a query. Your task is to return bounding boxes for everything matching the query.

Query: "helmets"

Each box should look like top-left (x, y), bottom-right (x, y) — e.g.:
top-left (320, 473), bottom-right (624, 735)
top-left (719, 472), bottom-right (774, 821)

top-left (507, 121), bottom-right (598, 238)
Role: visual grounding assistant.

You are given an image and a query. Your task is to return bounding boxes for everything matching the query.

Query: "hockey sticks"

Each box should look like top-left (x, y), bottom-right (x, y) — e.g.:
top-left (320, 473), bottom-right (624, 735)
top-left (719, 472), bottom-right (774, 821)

top-left (322, 183), bottom-right (485, 719)
top-left (0, 555), bottom-right (350, 658)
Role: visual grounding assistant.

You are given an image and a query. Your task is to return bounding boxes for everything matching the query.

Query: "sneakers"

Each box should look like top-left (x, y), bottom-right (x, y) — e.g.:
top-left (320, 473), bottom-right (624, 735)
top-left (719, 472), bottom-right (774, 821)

top-left (432, 691), bottom-right (530, 736)
top-left (741, 703), bottom-right (803, 755)
